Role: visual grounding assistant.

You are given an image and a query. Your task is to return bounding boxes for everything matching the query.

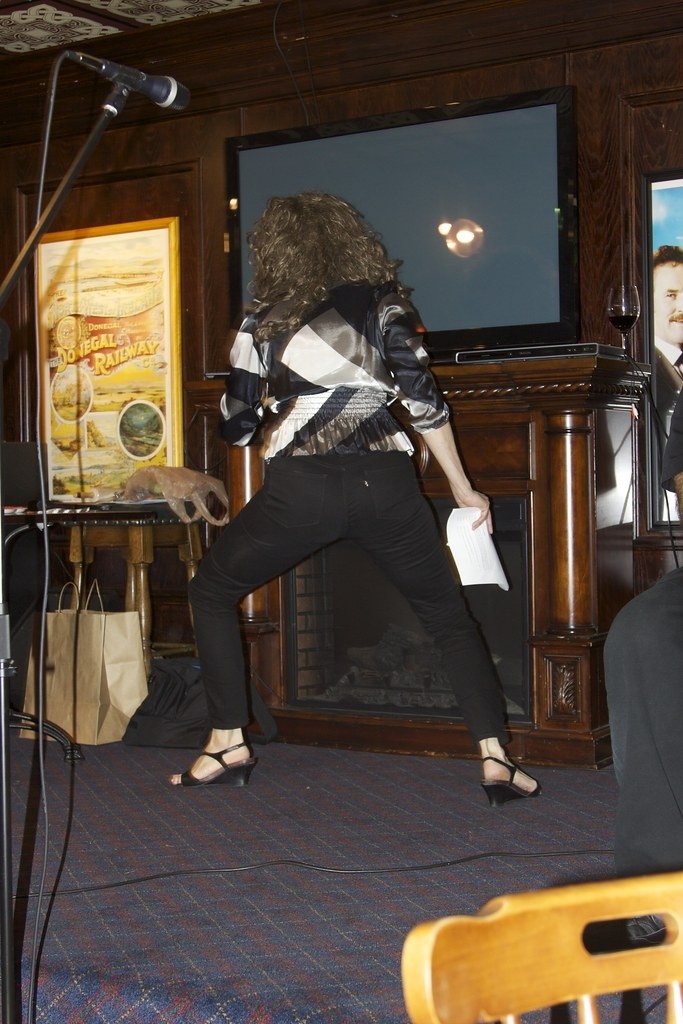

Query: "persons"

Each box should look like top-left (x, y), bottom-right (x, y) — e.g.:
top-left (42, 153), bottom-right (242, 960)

top-left (601, 378), bottom-right (683, 938)
top-left (170, 190), bottom-right (545, 807)
top-left (651, 244), bottom-right (683, 525)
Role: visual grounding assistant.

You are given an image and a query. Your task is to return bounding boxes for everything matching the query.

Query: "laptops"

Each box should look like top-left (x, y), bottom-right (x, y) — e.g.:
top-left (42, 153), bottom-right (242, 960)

top-left (2, 441), bottom-right (49, 506)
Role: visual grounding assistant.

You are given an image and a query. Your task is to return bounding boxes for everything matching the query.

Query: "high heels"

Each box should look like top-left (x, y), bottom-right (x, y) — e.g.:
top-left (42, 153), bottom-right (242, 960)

top-left (170, 743), bottom-right (258, 787)
top-left (481, 757), bottom-right (542, 808)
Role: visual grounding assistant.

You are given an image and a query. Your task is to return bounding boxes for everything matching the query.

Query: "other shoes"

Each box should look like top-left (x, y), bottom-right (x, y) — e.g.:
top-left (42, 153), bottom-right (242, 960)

top-left (578, 915), bottom-right (667, 955)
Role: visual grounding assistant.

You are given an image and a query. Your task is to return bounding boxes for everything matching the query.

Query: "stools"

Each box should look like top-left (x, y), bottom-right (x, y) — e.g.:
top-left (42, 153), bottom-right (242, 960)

top-left (58, 518), bottom-right (202, 682)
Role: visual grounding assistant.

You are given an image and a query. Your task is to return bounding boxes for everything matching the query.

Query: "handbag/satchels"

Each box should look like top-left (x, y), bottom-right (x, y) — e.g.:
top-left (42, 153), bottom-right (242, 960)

top-left (20, 578), bottom-right (148, 746)
top-left (121, 465), bottom-right (230, 527)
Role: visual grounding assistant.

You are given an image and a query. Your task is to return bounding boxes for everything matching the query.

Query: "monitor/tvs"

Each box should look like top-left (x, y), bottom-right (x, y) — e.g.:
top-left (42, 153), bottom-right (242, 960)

top-left (224, 86), bottom-right (579, 368)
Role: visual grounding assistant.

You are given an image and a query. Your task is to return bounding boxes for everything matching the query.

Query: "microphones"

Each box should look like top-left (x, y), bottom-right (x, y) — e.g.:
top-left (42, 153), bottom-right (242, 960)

top-left (64, 50), bottom-right (191, 111)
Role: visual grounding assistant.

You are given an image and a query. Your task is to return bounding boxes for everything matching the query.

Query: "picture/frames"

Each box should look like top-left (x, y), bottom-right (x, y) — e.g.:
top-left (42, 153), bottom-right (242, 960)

top-left (631, 168), bottom-right (683, 547)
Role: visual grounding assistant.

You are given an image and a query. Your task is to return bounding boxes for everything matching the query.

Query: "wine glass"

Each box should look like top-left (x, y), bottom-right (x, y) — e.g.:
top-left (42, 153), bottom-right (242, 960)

top-left (608, 286), bottom-right (641, 357)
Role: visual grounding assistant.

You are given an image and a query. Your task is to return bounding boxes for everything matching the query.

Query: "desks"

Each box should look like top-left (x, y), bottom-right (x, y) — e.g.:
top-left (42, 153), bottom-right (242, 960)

top-left (3, 507), bottom-right (158, 525)
